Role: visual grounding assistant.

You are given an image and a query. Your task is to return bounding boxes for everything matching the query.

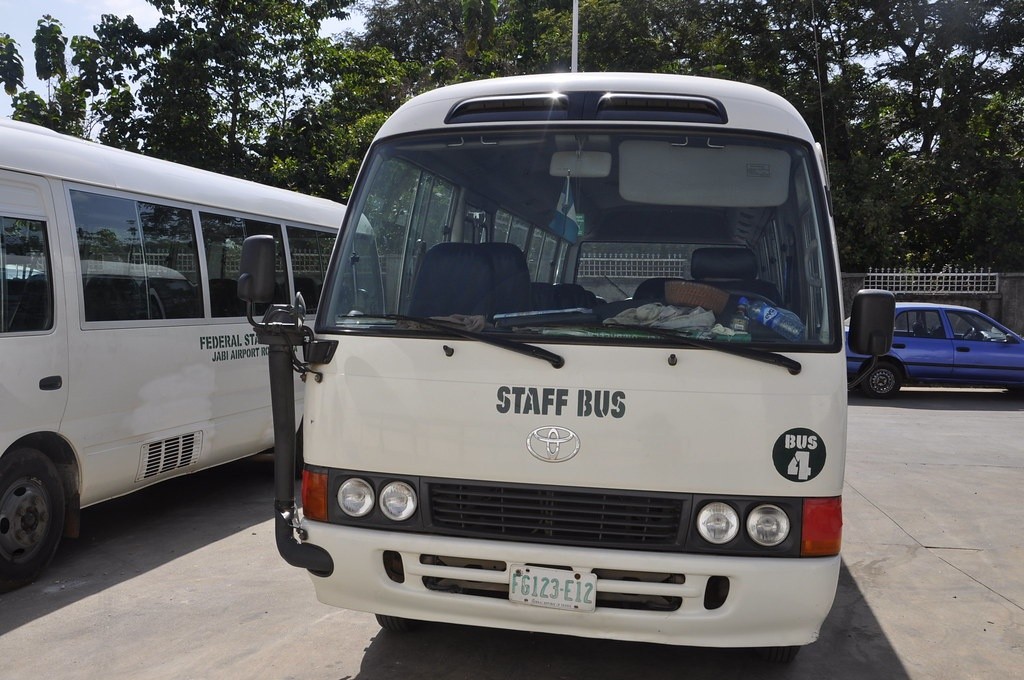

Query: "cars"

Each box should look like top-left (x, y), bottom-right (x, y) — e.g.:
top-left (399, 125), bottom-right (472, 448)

top-left (841, 301), bottom-right (1024, 400)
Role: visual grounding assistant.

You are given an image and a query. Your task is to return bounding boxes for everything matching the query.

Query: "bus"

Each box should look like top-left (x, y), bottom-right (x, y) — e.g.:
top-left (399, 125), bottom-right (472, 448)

top-left (236, 70), bottom-right (898, 665)
top-left (0, 117), bottom-right (382, 595)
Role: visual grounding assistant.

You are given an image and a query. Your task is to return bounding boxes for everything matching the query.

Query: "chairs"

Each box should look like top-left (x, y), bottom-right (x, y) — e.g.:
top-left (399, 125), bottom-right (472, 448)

top-left (532, 281), bottom-right (555, 310)
top-left (408, 242), bottom-right (496, 315)
top-left (480, 242), bottom-right (535, 314)
top-left (632, 278), bottom-right (687, 300)
top-left (690, 249), bottom-right (783, 307)
top-left (553, 282), bottom-right (587, 309)
top-left (5, 275), bottom-right (321, 330)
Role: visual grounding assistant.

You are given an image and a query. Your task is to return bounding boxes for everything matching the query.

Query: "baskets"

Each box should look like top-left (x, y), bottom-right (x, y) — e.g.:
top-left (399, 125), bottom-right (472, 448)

top-left (664, 281), bottom-right (730, 315)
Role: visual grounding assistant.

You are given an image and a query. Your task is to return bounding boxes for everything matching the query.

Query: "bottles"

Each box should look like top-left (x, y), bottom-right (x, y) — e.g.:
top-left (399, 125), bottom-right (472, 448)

top-left (729, 305), bottom-right (751, 333)
top-left (737, 296), bottom-right (806, 343)
